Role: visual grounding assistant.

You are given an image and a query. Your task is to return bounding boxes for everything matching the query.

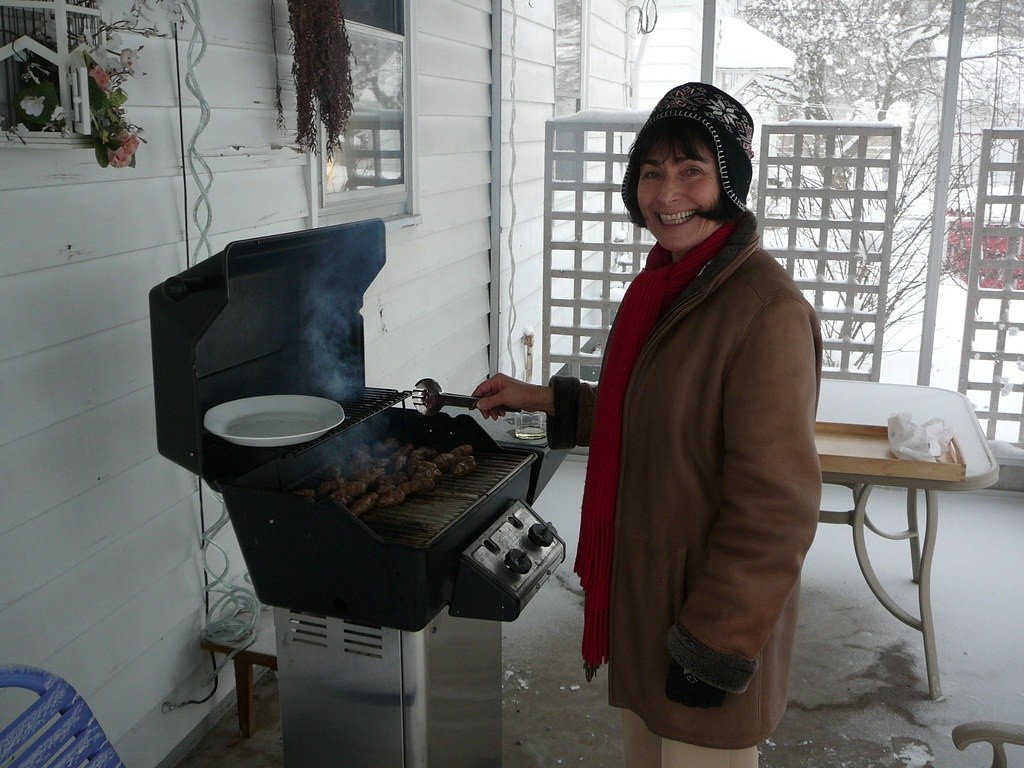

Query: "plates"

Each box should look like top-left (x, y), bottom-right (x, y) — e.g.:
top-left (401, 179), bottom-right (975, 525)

top-left (203, 395), bottom-right (346, 448)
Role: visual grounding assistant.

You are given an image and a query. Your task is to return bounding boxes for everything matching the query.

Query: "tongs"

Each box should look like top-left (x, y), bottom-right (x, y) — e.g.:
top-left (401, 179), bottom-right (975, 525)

top-left (411, 377), bottom-right (518, 416)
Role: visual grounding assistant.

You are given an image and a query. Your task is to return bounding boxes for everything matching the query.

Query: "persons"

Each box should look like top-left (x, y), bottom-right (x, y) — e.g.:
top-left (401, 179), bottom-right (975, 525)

top-left (468, 83), bottom-right (822, 767)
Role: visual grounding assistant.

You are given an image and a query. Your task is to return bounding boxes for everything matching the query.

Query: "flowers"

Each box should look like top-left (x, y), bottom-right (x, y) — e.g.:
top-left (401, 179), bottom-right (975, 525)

top-left (0, 0), bottom-right (186, 168)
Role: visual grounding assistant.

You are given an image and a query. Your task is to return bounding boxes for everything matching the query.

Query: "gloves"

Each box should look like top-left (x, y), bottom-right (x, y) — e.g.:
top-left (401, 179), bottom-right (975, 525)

top-left (664, 660), bottom-right (729, 707)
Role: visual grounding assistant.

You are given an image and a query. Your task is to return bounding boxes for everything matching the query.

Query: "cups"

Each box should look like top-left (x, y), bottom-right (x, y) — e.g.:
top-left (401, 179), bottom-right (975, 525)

top-left (514, 411), bottom-right (546, 440)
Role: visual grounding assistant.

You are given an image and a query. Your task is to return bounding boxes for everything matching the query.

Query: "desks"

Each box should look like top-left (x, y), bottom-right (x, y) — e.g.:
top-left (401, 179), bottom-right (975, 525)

top-left (816, 377), bottom-right (1000, 703)
top-left (200, 606), bottom-right (277, 738)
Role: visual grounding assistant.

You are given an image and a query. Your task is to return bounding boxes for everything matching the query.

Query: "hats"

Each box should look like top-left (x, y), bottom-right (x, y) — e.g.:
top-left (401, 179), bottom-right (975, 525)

top-left (620, 81), bottom-right (755, 213)
